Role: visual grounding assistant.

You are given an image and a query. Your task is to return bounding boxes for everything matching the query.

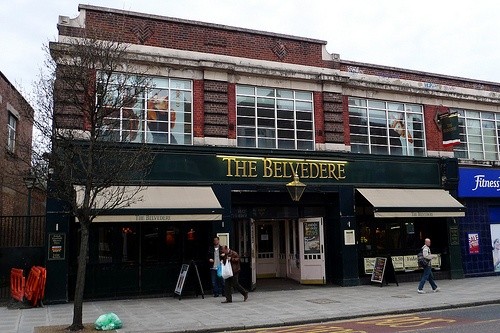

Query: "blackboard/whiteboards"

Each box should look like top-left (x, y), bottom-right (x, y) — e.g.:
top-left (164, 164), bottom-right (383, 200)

top-left (174, 259), bottom-right (204, 297)
top-left (371, 255), bottom-right (397, 284)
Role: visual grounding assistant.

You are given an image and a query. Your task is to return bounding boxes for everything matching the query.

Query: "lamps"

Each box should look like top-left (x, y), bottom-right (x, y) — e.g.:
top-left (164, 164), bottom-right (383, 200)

top-left (286, 169), bottom-right (305, 201)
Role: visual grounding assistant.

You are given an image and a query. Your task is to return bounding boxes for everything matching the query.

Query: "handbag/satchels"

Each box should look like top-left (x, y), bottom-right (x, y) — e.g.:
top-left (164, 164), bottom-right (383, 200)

top-left (217, 259), bottom-right (233, 279)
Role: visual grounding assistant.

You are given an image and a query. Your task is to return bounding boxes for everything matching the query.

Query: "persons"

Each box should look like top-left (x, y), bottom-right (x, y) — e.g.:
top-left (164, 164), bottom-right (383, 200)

top-left (492, 238), bottom-right (500, 273)
top-left (218, 245), bottom-right (248, 303)
top-left (207, 236), bottom-right (228, 297)
top-left (417, 238), bottom-right (439, 293)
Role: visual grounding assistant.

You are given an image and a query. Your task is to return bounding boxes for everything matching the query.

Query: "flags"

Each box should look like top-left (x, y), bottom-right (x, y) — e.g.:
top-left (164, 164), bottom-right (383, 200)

top-left (439, 111), bottom-right (461, 147)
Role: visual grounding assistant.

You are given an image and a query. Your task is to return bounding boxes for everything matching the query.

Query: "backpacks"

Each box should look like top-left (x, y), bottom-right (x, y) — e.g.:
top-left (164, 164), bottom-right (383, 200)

top-left (417, 248), bottom-right (431, 268)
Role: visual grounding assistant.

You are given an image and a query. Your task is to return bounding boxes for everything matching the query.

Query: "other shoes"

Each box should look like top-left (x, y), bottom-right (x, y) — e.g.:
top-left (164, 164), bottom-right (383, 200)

top-left (243, 293), bottom-right (248, 302)
top-left (433, 287), bottom-right (440, 293)
top-left (418, 289), bottom-right (426, 293)
top-left (221, 300), bottom-right (232, 303)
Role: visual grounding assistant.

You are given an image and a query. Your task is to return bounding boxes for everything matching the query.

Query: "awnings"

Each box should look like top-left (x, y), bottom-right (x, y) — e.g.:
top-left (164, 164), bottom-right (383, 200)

top-left (355, 186), bottom-right (467, 219)
top-left (70, 184), bottom-right (224, 223)
